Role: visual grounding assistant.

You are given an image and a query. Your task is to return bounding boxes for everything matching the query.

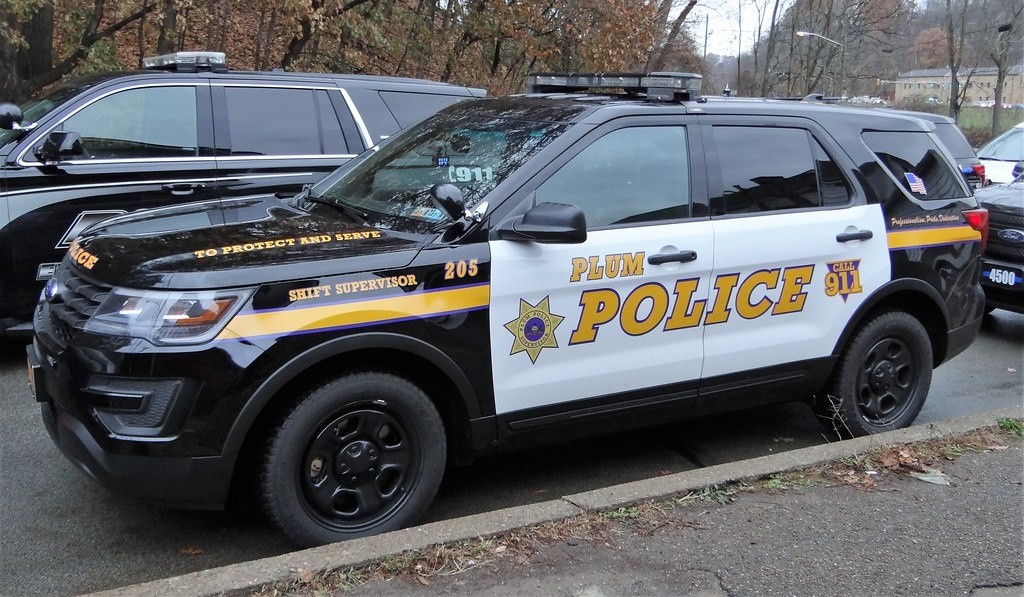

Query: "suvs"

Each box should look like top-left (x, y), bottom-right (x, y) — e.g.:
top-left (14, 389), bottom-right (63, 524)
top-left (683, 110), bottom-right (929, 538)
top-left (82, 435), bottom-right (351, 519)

top-left (868, 103), bottom-right (1024, 323)
top-left (0, 48), bottom-right (489, 355)
top-left (23, 70), bottom-right (995, 548)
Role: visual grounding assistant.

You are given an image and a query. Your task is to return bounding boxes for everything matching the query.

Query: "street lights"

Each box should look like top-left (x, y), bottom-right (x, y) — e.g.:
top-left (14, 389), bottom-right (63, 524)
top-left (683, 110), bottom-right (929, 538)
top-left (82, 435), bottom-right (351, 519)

top-left (796, 30), bottom-right (845, 102)
